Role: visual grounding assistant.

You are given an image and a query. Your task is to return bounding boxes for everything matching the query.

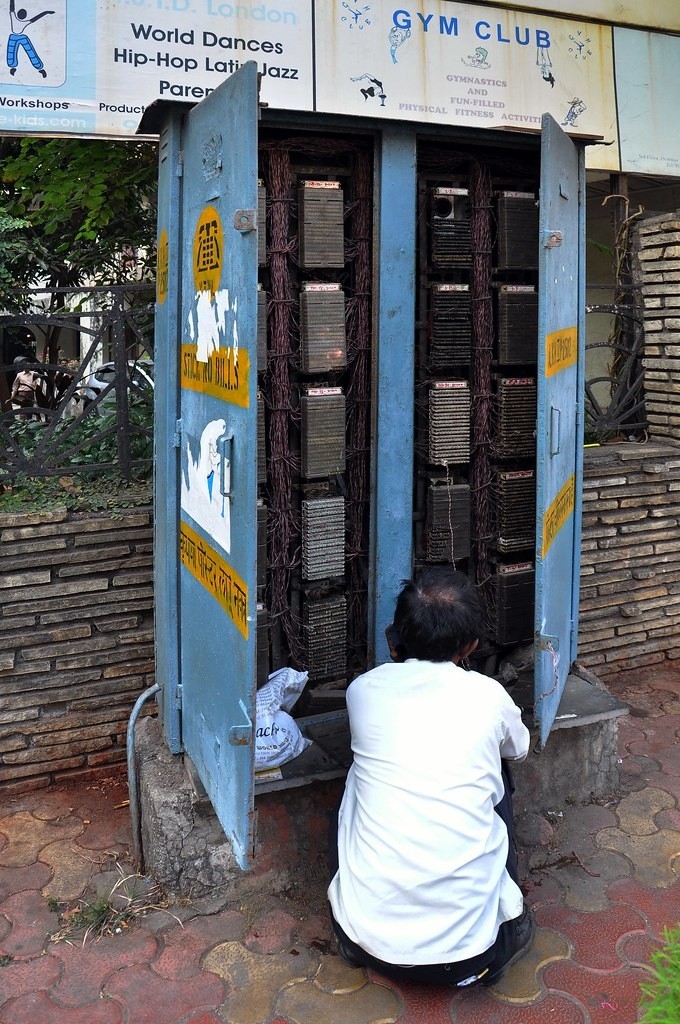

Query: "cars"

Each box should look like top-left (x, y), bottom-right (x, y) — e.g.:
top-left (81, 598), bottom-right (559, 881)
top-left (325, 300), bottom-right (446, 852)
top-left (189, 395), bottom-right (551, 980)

top-left (82, 358), bottom-right (154, 419)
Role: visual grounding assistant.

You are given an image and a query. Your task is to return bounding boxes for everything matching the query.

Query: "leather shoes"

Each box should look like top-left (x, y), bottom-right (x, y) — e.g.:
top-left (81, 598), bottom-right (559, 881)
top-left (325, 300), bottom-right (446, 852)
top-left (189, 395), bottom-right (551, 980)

top-left (479, 915), bottom-right (537, 986)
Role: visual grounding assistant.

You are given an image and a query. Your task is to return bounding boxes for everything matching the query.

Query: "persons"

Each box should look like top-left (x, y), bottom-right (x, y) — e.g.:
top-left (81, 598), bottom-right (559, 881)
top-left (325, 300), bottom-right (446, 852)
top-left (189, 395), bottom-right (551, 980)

top-left (10, 346), bottom-right (86, 419)
top-left (325, 571), bottom-right (537, 989)
